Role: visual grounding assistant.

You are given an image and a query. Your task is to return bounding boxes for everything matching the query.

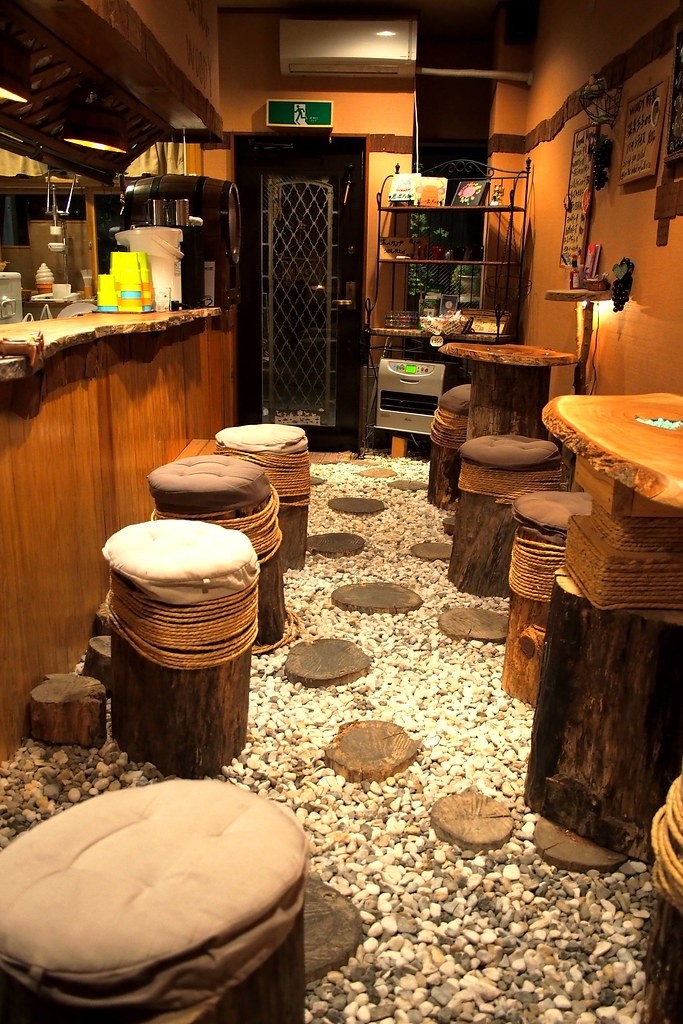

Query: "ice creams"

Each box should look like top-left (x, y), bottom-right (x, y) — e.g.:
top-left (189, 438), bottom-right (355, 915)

top-left (35, 262), bottom-right (54, 293)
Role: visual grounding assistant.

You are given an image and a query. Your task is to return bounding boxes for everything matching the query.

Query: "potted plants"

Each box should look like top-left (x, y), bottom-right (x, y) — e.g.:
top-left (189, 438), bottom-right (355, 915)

top-left (451, 264), bottom-right (480, 294)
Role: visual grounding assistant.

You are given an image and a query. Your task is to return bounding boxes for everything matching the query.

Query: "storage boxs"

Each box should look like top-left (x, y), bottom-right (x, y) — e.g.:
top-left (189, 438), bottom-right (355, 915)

top-left (450, 180), bottom-right (490, 205)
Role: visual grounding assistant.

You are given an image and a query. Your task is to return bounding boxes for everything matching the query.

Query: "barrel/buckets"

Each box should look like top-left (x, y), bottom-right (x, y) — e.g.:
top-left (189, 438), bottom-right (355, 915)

top-left (124, 174), bottom-right (241, 265)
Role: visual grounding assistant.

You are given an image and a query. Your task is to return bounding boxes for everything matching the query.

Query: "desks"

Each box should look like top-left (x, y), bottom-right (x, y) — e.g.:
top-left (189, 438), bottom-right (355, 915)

top-left (439, 344), bottom-right (578, 442)
top-left (524, 393), bottom-right (683, 863)
top-left (544, 288), bottom-right (612, 395)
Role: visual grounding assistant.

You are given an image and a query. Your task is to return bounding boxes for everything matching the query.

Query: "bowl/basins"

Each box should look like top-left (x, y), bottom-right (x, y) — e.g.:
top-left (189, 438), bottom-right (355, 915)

top-left (585, 278), bottom-right (608, 291)
top-left (146, 198), bottom-right (189, 227)
top-left (48, 242), bottom-right (65, 252)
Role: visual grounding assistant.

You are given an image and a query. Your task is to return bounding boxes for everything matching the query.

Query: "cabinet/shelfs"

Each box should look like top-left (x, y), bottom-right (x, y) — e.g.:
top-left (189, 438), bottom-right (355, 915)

top-left (357, 157), bottom-right (532, 459)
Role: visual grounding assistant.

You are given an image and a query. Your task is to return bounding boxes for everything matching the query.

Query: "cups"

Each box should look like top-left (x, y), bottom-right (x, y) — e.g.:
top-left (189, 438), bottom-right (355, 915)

top-left (22, 290), bottom-right (32, 301)
top-left (51, 283), bottom-right (71, 299)
top-left (97, 252), bottom-right (155, 313)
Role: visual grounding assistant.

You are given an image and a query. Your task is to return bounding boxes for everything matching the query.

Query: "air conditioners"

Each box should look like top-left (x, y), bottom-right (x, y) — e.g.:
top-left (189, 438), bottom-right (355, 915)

top-left (280, 19), bottom-right (418, 78)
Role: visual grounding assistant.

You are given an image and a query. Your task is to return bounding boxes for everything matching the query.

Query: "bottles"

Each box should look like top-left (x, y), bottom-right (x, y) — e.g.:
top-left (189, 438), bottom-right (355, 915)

top-left (570, 254), bottom-right (580, 289)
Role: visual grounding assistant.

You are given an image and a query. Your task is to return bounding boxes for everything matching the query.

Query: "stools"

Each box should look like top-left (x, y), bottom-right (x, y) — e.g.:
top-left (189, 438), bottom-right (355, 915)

top-left (0, 779), bottom-right (306, 1024)
top-left (447, 435), bottom-right (566, 597)
top-left (148, 455), bottom-right (285, 646)
top-left (502, 492), bottom-right (593, 707)
top-left (215, 424), bottom-right (310, 571)
top-left (428, 384), bottom-right (471, 510)
top-left (100, 519), bottom-right (260, 779)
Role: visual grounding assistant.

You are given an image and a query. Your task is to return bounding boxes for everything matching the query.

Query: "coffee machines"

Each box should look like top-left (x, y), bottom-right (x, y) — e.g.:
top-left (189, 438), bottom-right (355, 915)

top-left (115, 225), bottom-right (184, 312)
